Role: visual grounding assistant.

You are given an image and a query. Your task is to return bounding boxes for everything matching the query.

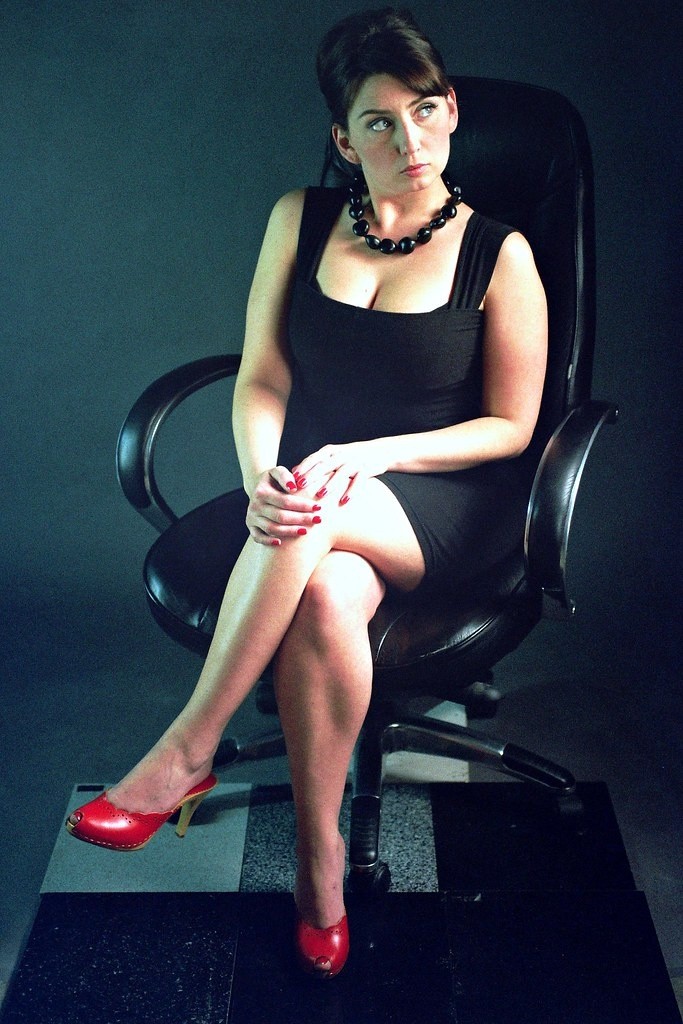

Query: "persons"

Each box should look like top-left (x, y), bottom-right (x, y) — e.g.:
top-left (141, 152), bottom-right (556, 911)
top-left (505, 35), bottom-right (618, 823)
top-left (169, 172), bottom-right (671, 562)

top-left (65, 6), bottom-right (550, 981)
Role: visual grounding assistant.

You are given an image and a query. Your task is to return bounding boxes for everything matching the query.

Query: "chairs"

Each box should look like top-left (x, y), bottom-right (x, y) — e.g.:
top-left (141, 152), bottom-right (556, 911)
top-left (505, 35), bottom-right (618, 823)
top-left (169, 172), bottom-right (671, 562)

top-left (114, 76), bottom-right (615, 960)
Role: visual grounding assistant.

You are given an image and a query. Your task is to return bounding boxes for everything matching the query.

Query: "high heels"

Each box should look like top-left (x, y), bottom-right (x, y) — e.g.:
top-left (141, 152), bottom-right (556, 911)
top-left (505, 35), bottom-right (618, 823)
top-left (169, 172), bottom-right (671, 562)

top-left (65, 771), bottom-right (219, 854)
top-left (297, 849), bottom-right (352, 976)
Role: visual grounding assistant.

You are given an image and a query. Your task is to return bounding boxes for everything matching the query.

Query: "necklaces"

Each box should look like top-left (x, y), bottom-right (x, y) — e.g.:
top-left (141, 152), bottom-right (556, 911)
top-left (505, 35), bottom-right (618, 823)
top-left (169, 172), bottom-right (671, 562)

top-left (350, 177), bottom-right (462, 254)
